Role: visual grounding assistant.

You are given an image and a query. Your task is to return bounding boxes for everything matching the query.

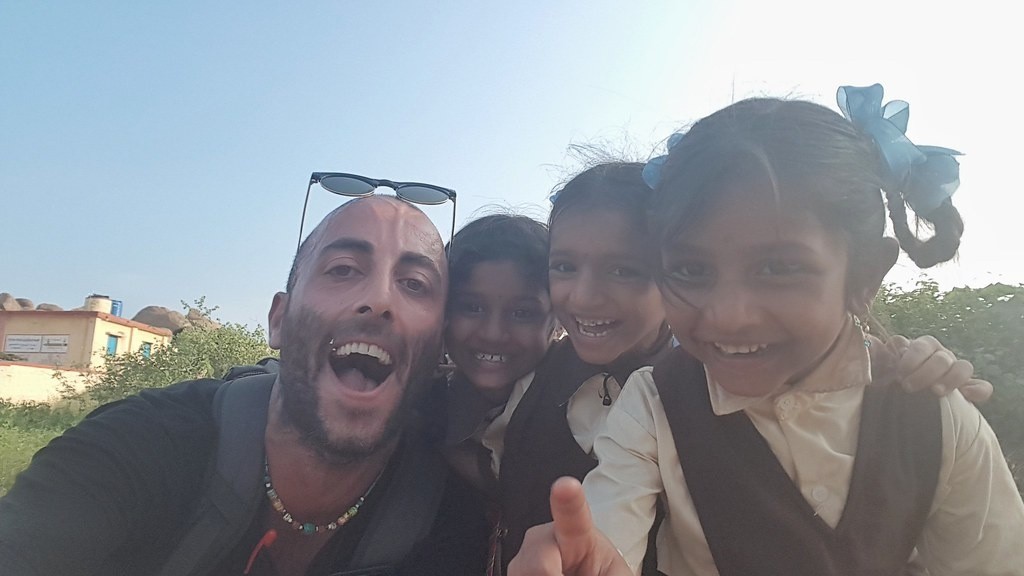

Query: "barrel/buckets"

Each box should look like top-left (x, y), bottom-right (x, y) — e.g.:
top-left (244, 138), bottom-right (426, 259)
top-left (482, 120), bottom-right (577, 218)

top-left (111, 300), bottom-right (122, 316)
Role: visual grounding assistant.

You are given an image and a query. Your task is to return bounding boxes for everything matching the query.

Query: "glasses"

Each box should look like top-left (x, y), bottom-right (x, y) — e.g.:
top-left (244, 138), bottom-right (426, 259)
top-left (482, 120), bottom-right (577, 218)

top-left (293, 171), bottom-right (457, 274)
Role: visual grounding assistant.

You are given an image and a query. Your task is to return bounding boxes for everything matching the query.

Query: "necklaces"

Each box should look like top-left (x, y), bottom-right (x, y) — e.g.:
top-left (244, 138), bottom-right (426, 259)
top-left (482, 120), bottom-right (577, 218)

top-left (264, 458), bottom-right (389, 533)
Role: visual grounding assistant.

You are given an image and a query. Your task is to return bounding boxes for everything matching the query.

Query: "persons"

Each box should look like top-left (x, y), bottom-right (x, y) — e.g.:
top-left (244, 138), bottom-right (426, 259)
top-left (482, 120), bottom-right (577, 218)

top-left (433, 214), bottom-right (563, 522)
top-left (507, 84), bottom-right (1023, 576)
top-left (488, 160), bottom-right (994, 575)
top-left (0, 172), bottom-right (494, 576)
